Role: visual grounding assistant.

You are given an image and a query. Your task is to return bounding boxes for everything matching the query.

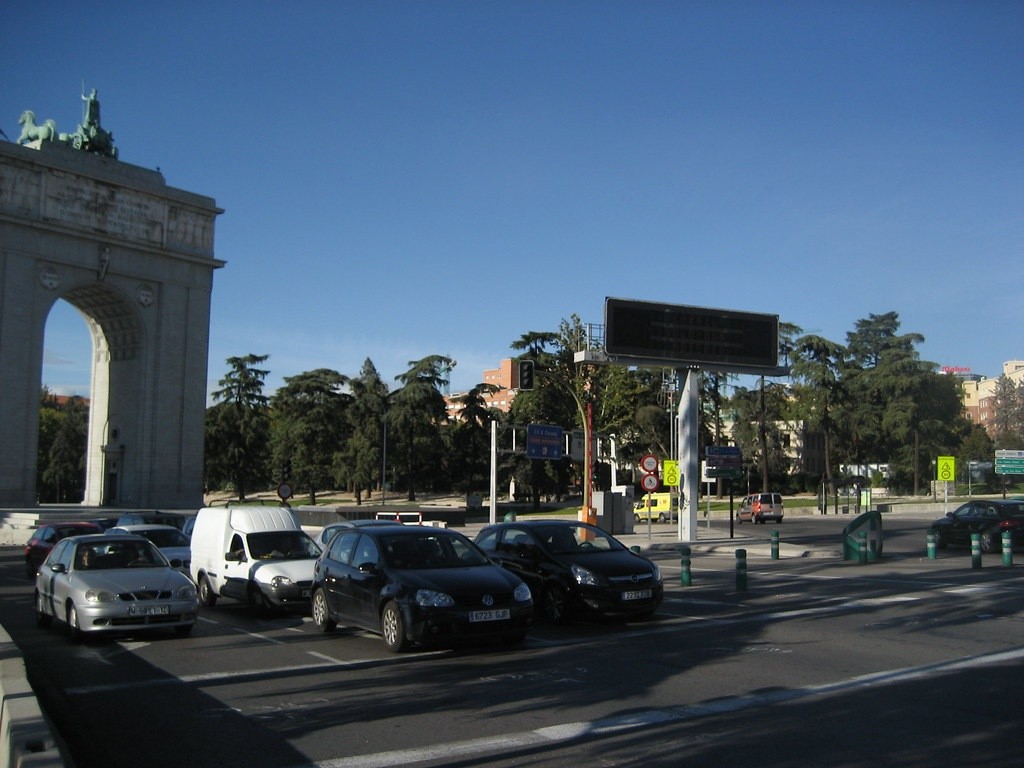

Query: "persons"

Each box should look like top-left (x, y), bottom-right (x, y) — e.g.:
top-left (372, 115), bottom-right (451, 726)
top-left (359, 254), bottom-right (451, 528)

top-left (424, 544), bottom-right (445, 565)
top-left (77, 550), bottom-right (97, 569)
top-left (520, 535), bottom-right (537, 559)
top-left (82, 87), bottom-right (100, 131)
top-left (130, 550), bottom-right (144, 563)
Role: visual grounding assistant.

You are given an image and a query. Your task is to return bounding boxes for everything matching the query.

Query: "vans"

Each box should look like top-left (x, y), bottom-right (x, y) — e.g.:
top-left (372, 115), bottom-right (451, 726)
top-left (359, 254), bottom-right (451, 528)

top-left (634, 492), bottom-right (678, 523)
top-left (735, 493), bottom-right (784, 524)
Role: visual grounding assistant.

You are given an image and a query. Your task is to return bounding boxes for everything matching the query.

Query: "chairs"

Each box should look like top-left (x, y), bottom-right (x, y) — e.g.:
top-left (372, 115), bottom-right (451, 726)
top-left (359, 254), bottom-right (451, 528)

top-left (414, 540), bottom-right (442, 568)
top-left (513, 534), bottom-right (527, 550)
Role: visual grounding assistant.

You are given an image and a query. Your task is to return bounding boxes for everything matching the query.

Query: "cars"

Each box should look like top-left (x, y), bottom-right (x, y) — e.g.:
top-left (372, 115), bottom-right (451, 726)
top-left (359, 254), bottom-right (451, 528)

top-left (927, 499), bottom-right (1024, 554)
top-left (305, 526), bottom-right (534, 653)
top-left (475, 519), bottom-right (664, 626)
top-left (316, 518), bottom-right (404, 556)
top-left (25, 498), bottom-right (324, 633)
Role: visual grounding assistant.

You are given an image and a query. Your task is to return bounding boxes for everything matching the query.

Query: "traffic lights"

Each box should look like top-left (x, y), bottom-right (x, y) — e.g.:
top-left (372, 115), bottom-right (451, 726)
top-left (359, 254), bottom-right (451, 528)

top-left (519, 360), bottom-right (535, 391)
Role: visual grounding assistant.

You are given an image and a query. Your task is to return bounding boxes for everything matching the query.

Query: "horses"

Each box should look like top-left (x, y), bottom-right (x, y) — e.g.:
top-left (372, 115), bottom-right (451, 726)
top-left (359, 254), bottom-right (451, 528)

top-left (17, 110), bottom-right (56, 150)
